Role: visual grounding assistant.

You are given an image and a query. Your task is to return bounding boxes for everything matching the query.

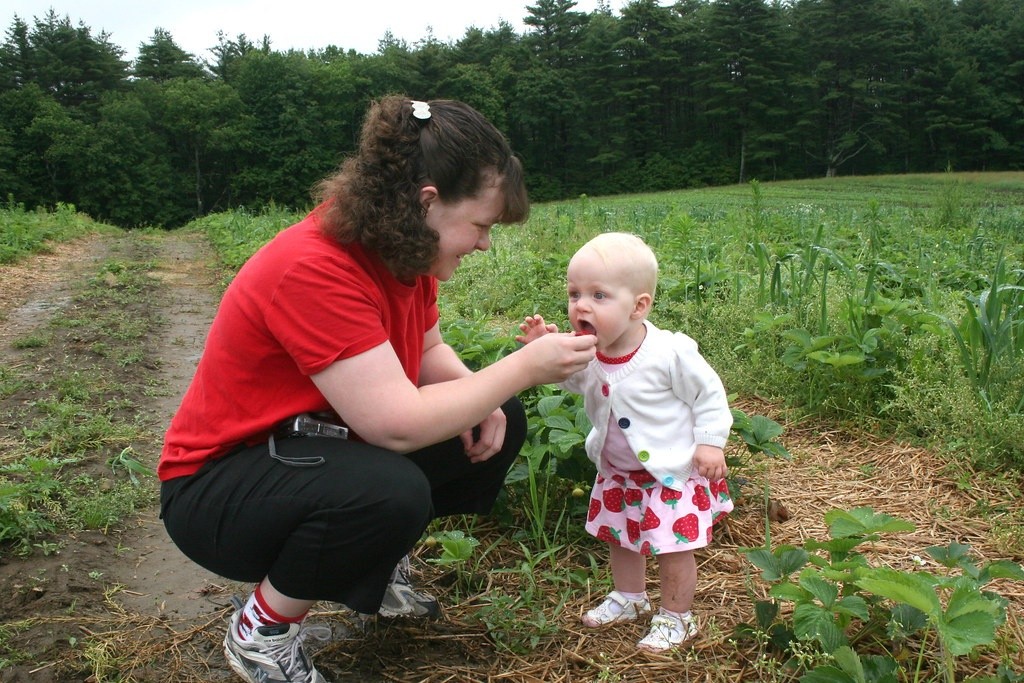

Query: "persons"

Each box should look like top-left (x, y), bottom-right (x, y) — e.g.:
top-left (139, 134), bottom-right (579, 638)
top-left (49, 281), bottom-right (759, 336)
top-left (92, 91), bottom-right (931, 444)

top-left (157, 97), bottom-right (597, 683)
top-left (515, 232), bottom-right (733, 654)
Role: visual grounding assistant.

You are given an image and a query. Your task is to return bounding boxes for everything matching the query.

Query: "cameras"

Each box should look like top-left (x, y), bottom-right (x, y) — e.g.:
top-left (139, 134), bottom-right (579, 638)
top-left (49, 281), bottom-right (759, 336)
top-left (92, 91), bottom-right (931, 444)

top-left (273, 412), bottom-right (349, 442)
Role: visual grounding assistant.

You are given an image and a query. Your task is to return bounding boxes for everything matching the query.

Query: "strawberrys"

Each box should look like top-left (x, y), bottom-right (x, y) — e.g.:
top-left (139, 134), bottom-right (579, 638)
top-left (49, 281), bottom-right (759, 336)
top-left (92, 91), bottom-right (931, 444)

top-left (575, 329), bottom-right (594, 336)
top-left (591, 472), bottom-right (731, 556)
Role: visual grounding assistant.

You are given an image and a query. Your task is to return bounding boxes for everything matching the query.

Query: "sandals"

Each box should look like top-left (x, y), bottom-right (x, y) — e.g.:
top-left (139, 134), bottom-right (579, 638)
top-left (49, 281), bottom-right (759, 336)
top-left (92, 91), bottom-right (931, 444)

top-left (582, 591), bottom-right (651, 627)
top-left (636, 614), bottom-right (698, 653)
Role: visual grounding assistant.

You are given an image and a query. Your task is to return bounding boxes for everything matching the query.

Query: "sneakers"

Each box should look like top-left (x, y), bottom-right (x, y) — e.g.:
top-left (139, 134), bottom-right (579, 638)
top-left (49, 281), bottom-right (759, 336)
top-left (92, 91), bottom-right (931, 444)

top-left (360, 555), bottom-right (439, 621)
top-left (222, 596), bottom-right (333, 683)
